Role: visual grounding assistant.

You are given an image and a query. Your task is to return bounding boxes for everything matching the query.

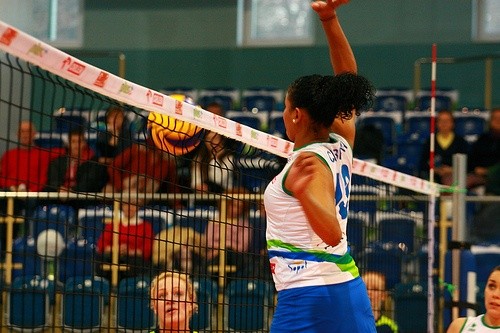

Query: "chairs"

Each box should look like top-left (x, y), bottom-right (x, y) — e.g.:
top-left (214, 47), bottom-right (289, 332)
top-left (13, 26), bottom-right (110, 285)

top-left (5, 87), bottom-right (500, 333)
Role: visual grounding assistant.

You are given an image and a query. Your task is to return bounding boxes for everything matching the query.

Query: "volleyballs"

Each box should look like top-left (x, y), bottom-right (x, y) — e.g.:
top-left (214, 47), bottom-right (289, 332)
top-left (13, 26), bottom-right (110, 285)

top-left (148, 95), bottom-right (206, 153)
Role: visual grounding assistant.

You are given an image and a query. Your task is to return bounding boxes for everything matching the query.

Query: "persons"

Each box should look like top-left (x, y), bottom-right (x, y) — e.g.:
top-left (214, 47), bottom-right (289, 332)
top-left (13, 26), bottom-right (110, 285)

top-left (446, 265), bottom-right (499, 333)
top-left (263, 0), bottom-right (376, 333)
top-left (357, 271), bottom-right (398, 333)
top-left (469, 109), bottom-right (500, 196)
top-left (423, 112), bottom-right (472, 185)
top-left (150, 271), bottom-right (199, 332)
top-left (0, 104), bottom-right (252, 277)
